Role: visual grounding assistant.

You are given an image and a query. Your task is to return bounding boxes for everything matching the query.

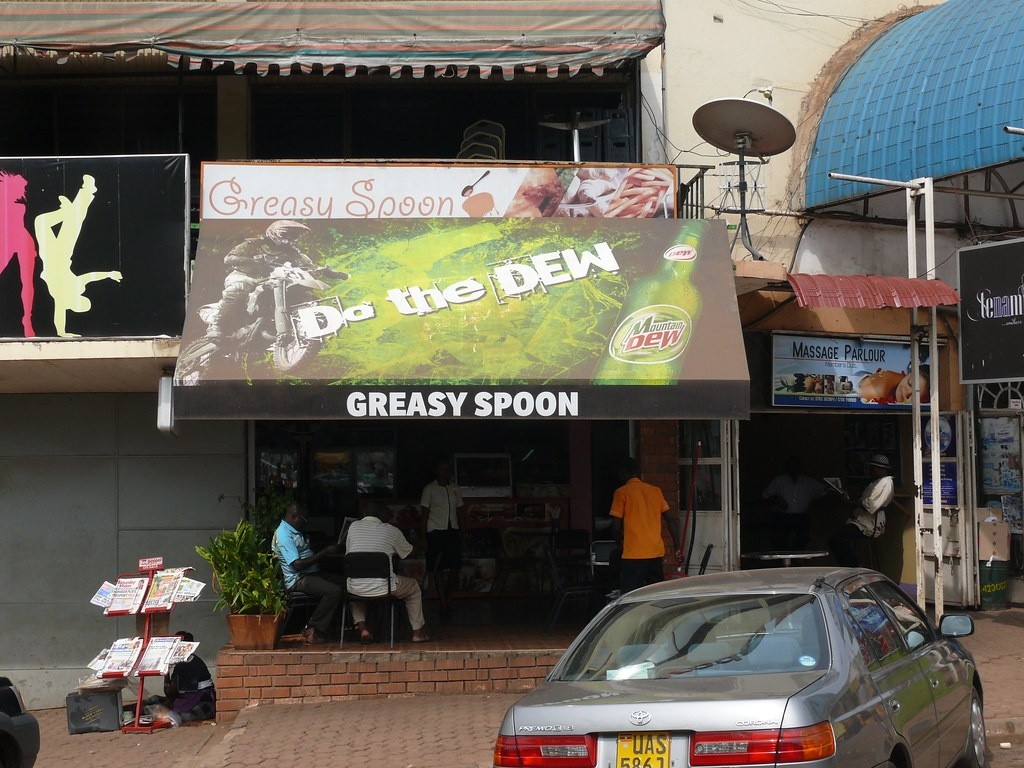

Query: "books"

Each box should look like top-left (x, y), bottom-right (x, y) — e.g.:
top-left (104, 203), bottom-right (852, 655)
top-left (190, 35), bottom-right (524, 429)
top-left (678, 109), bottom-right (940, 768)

top-left (86, 564), bottom-right (207, 676)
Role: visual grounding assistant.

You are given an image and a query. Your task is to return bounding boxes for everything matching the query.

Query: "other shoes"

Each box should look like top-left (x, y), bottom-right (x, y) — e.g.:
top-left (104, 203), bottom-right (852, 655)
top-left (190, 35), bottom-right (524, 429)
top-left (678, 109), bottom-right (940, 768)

top-left (301, 627), bottom-right (320, 644)
top-left (316, 630), bottom-right (327, 643)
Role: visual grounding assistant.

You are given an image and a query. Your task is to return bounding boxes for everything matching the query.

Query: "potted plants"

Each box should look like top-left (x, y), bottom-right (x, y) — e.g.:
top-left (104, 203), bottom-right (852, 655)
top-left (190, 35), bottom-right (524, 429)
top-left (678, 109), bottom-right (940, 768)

top-left (194, 517), bottom-right (288, 650)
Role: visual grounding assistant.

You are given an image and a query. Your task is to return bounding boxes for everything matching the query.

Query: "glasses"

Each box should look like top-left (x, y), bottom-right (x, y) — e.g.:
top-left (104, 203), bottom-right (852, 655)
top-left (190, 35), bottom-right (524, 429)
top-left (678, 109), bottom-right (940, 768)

top-left (295, 511), bottom-right (309, 524)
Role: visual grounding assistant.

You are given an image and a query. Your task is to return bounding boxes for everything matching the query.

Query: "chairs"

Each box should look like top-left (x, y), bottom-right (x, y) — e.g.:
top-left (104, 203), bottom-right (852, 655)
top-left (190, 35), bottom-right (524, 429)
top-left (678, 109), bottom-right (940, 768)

top-left (748, 636), bottom-right (802, 671)
top-left (271, 552), bottom-right (356, 647)
top-left (589, 540), bottom-right (623, 600)
top-left (538, 544), bottom-right (607, 633)
top-left (334, 552), bottom-right (400, 649)
top-left (616, 643), bottom-right (672, 670)
top-left (687, 643), bottom-right (733, 676)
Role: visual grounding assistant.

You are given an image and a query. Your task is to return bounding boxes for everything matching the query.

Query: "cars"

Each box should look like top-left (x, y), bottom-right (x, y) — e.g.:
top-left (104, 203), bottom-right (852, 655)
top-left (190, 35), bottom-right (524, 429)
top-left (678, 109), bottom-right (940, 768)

top-left (491, 566), bottom-right (988, 768)
top-left (0, 676), bottom-right (40, 768)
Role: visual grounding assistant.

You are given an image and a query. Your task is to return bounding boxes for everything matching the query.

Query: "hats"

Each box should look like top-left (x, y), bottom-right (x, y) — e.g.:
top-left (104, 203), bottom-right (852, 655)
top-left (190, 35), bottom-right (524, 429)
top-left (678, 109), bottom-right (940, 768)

top-left (864, 455), bottom-right (893, 469)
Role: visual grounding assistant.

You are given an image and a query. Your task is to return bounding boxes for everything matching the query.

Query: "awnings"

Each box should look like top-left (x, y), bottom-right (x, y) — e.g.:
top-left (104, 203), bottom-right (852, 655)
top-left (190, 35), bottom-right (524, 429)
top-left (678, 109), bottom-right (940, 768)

top-left (172, 215), bottom-right (753, 420)
top-left (0, 1), bottom-right (665, 79)
top-left (786, 270), bottom-right (960, 309)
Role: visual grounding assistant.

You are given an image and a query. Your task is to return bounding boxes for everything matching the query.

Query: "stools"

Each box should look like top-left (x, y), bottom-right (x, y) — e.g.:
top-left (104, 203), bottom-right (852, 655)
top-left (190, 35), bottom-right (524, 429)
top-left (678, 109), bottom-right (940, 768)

top-left (859, 536), bottom-right (881, 570)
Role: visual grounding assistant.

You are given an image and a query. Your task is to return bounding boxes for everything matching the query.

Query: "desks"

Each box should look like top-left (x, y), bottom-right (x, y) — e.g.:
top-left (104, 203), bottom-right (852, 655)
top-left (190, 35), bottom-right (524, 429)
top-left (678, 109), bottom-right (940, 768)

top-left (742, 550), bottom-right (830, 567)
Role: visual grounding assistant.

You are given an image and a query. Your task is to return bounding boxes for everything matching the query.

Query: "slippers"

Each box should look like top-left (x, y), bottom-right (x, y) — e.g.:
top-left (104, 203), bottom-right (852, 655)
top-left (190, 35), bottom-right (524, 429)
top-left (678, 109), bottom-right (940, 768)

top-left (362, 634), bottom-right (374, 644)
top-left (411, 633), bottom-right (430, 642)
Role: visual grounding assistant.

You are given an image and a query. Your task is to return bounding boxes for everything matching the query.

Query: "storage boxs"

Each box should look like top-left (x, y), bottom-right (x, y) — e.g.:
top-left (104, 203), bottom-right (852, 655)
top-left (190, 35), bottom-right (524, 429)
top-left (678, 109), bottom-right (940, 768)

top-left (66, 690), bottom-right (124, 735)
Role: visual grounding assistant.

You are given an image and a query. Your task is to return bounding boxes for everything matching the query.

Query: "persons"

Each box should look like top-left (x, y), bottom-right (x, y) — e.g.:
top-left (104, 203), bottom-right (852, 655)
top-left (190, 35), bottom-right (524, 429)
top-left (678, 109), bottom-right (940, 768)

top-left (271, 501), bottom-right (347, 643)
top-left (203, 218), bottom-right (344, 348)
top-left (344, 499), bottom-right (430, 643)
top-left (122, 631), bottom-right (216, 723)
top-left (419, 458), bottom-right (464, 621)
top-left (760, 455), bottom-right (828, 552)
top-left (610, 456), bottom-right (669, 596)
top-left (827, 453), bottom-right (894, 566)
top-left (786, 371), bottom-right (855, 395)
top-left (859, 364), bottom-right (931, 404)
